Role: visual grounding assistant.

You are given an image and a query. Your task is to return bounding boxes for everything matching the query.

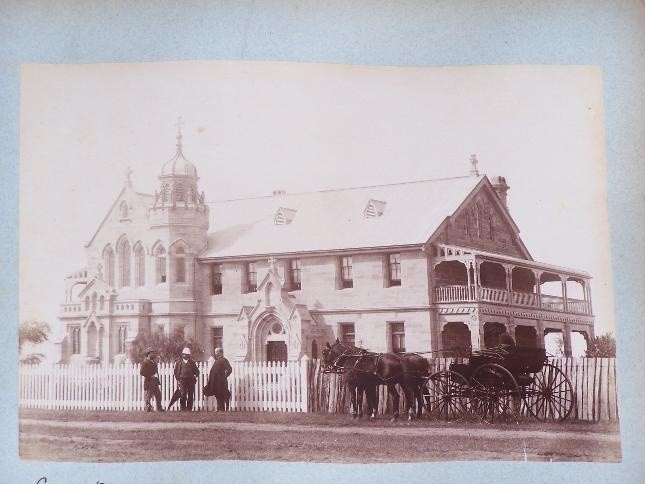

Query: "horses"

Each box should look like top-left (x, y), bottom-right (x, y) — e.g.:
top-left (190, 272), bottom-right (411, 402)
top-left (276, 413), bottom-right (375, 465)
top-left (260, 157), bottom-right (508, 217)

top-left (320, 337), bottom-right (431, 424)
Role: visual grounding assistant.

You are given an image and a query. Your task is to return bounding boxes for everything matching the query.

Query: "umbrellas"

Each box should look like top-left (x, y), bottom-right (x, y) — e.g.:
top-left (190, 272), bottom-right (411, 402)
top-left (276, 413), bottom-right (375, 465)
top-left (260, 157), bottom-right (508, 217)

top-left (166, 389), bottom-right (181, 412)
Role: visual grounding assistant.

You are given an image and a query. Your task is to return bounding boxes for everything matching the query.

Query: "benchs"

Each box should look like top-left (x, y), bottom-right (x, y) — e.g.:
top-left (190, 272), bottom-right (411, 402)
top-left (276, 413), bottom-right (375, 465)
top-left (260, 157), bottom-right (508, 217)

top-left (469, 346), bottom-right (546, 374)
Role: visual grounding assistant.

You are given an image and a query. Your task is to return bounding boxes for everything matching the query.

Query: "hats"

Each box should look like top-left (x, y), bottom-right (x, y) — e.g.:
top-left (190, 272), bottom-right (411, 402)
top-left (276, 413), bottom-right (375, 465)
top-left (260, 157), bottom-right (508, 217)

top-left (181, 346), bottom-right (191, 354)
top-left (144, 346), bottom-right (154, 353)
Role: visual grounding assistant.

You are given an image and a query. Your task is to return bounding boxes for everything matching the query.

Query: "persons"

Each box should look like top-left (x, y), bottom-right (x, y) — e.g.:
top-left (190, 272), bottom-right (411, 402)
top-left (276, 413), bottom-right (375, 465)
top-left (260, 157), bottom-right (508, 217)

top-left (140, 346), bottom-right (165, 413)
top-left (210, 347), bottom-right (232, 411)
top-left (174, 348), bottom-right (199, 412)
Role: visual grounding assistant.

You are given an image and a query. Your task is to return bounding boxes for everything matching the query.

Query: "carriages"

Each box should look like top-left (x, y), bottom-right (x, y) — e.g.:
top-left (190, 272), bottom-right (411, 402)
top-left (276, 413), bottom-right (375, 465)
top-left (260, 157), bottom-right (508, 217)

top-left (318, 337), bottom-right (575, 427)
